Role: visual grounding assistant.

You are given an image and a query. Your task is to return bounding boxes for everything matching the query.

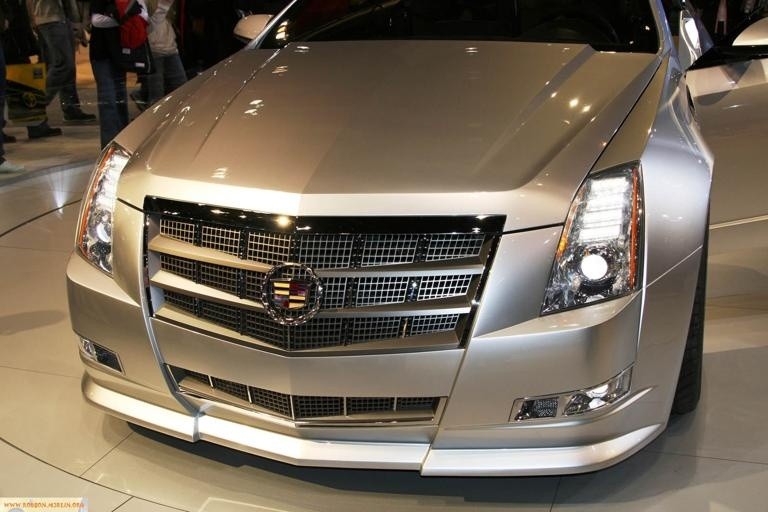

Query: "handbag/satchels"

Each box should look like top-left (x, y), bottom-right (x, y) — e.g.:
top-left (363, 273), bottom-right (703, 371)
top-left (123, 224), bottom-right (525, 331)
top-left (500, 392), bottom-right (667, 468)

top-left (2, 62), bottom-right (48, 127)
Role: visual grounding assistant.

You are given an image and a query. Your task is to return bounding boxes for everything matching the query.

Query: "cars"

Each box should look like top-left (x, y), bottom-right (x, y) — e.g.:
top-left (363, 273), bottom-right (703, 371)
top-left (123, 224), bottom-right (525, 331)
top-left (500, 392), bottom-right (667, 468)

top-left (63, 3), bottom-right (768, 480)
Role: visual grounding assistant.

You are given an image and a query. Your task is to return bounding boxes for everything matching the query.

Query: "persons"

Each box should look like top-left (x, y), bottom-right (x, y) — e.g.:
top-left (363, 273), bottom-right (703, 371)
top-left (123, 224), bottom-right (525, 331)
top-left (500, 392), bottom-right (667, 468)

top-left (2, 1), bottom-right (96, 143)
top-left (89, 1), bottom-right (277, 150)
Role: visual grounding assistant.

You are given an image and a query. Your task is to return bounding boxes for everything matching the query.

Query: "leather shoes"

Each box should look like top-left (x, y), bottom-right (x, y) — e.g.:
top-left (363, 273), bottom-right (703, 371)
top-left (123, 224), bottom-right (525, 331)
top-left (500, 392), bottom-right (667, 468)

top-left (25, 124), bottom-right (64, 140)
top-left (61, 111), bottom-right (98, 124)
top-left (1, 131), bottom-right (17, 144)
